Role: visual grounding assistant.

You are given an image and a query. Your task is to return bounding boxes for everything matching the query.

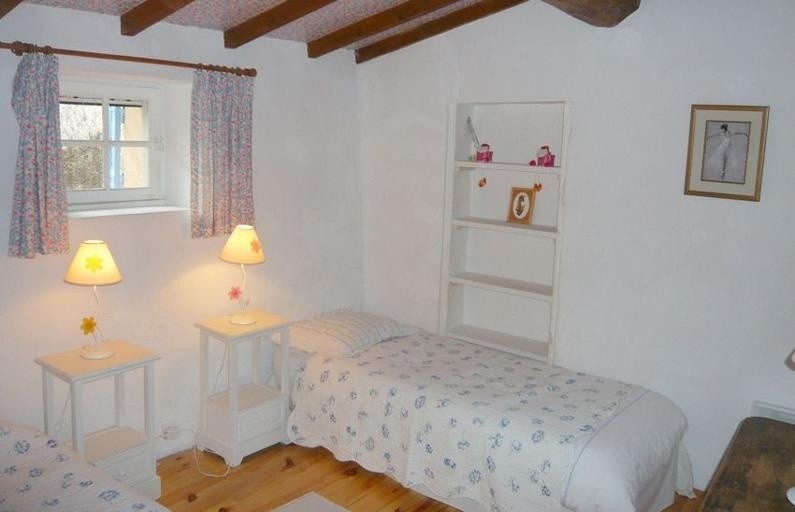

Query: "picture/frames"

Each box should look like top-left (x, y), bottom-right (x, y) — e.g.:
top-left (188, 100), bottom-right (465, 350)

top-left (506, 187), bottom-right (536, 224)
top-left (684, 104), bottom-right (770, 203)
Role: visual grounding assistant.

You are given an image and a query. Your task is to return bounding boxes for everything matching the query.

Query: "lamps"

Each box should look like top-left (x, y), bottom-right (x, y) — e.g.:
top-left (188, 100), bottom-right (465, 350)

top-left (219, 224), bottom-right (265, 327)
top-left (64, 239), bottom-right (123, 360)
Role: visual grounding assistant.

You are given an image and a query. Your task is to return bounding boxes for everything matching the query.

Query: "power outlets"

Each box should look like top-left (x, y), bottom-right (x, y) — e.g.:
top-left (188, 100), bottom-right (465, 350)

top-left (161, 423), bottom-right (176, 440)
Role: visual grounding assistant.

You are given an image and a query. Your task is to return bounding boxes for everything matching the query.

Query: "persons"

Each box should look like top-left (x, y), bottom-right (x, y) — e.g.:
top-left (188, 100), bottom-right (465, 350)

top-left (708, 125), bottom-right (749, 181)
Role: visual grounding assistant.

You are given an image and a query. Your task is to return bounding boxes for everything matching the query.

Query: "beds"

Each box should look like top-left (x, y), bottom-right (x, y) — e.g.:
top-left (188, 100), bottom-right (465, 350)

top-left (0, 421), bottom-right (171, 511)
top-left (266, 308), bottom-right (686, 512)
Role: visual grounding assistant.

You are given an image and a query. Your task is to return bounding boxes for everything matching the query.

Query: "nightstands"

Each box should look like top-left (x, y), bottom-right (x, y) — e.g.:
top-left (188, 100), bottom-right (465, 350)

top-left (34, 340), bottom-right (163, 501)
top-left (192, 309), bottom-right (295, 467)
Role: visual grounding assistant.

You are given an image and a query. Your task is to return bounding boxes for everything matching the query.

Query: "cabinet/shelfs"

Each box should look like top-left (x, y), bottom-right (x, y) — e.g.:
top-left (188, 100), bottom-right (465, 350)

top-left (439, 97), bottom-right (571, 366)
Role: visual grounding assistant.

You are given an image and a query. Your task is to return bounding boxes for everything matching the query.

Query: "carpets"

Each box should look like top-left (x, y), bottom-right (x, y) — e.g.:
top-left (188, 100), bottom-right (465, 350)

top-left (268, 489), bottom-right (351, 512)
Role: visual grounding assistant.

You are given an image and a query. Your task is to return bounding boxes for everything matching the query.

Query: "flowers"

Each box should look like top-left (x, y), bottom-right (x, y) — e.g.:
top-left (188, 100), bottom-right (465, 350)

top-left (80, 316), bottom-right (97, 336)
top-left (227, 286), bottom-right (243, 300)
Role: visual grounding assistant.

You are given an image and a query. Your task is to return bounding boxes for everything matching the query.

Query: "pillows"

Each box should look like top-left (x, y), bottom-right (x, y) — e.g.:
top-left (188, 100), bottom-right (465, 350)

top-left (269, 309), bottom-right (422, 359)
